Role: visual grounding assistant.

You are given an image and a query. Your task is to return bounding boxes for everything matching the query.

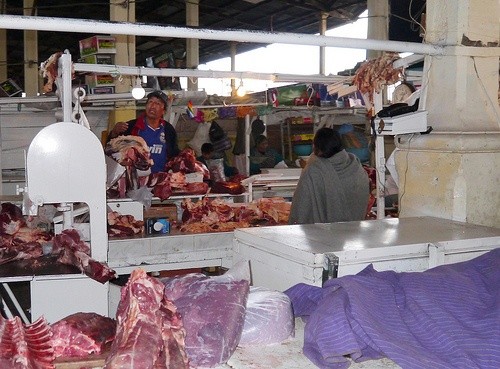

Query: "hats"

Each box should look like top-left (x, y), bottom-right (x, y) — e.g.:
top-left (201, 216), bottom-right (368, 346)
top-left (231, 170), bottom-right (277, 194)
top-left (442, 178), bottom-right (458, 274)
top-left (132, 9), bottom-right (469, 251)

top-left (147, 91), bottom-right (168, 104)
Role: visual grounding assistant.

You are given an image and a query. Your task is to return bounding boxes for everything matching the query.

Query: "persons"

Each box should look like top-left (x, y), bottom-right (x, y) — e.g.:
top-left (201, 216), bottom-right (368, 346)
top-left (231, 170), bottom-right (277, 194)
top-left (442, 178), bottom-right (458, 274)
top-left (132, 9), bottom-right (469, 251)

top-left (233, 119), bottom-right (271, 154)
top-left (105, 89), bottom-right (179, 172)
top-left (196, 143), bottom-right (238, 176)
top-left (336, 125), bottom-right (370, 162)
top-left (250, 134), bottom-right (296, 175)
top-left (288, 126), bottom-right (370, 223)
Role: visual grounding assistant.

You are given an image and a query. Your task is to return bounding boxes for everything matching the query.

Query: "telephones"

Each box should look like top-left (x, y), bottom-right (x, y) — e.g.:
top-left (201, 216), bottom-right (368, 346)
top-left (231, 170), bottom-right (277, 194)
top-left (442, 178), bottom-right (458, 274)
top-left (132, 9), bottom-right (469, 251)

top-left (379, 102), bottom-right (416, 118)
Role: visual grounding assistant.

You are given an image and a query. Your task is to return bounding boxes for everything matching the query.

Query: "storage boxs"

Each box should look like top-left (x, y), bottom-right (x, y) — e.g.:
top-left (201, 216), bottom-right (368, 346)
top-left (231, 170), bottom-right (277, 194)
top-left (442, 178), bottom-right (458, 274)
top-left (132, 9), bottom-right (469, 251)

top-left (78, 36), bottom-right (117, 106)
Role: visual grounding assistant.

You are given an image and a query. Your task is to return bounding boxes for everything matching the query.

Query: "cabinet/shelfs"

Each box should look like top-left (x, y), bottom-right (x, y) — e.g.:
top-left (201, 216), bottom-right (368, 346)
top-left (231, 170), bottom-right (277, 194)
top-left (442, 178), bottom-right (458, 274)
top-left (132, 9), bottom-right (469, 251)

top-left (280, 116), bottom-right (315, 163)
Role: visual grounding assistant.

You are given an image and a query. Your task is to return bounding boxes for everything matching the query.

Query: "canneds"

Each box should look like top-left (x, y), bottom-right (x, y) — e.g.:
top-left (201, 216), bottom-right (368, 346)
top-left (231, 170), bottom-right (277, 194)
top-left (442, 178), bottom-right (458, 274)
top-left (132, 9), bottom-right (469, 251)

top-left (268, 88), bottom-right (278, 108)
